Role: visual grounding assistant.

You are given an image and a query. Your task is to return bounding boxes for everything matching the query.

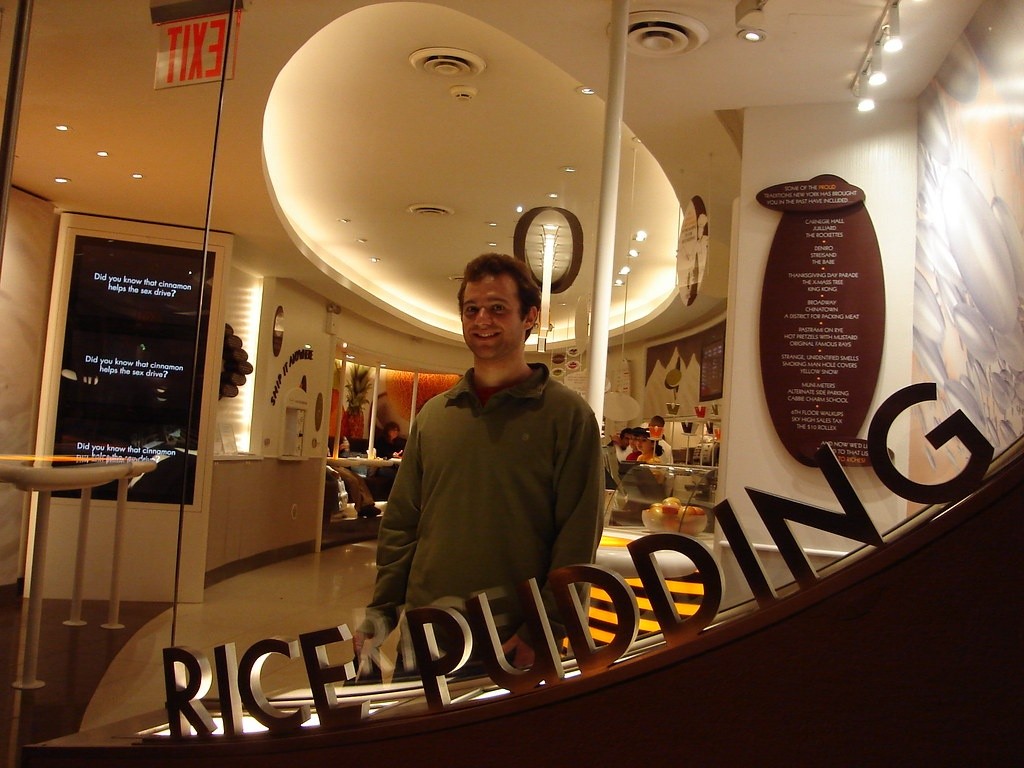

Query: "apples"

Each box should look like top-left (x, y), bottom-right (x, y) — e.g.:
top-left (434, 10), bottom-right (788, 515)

top-left (644, 497), bottom-right (705, 535)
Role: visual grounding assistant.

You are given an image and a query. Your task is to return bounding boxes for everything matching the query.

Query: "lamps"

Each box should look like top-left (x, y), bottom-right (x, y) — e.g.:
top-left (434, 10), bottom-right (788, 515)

top-left (882, 3), bottom-right (903, 52)
top-left (857, 74), bottom-right (875, 112)
top-left (868, 45), bottom-right (886, 86)
top-left (536, 223), bottom-right (562, 353)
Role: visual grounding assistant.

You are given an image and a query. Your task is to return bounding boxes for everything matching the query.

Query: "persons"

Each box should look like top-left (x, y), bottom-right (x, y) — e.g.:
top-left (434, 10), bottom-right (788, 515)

top-left (353, 253), bottom-right (606, 670)
top-left (612, 427), bottom-right (673, 463)
top-left (327, 422), bottom-right (404, 518)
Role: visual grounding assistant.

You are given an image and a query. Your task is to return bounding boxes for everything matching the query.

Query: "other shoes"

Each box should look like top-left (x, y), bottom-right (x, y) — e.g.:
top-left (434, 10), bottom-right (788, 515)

top-left (357, 504), bottom-right (381, 519)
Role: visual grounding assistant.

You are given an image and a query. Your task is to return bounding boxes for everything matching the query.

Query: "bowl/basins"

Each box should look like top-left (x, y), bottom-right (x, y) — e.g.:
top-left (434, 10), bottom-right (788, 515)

top-left (642, 510), bottom-right (707, 535)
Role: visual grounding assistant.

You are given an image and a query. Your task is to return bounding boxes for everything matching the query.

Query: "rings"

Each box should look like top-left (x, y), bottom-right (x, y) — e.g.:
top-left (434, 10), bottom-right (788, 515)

top-left (516, 664), bottom-right (532, 669)
